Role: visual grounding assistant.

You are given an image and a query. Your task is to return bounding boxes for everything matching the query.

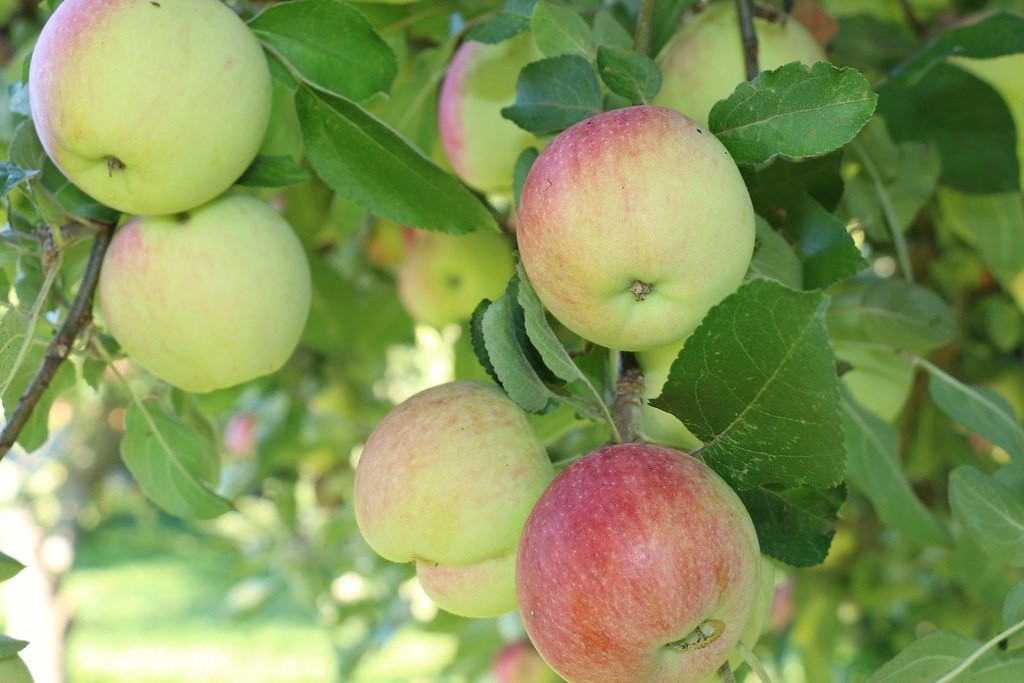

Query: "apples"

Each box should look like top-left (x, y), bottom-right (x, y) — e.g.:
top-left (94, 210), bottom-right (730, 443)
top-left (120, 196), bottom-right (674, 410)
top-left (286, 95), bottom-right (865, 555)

top-left (30, 0), bottom-right (1024, 683)
